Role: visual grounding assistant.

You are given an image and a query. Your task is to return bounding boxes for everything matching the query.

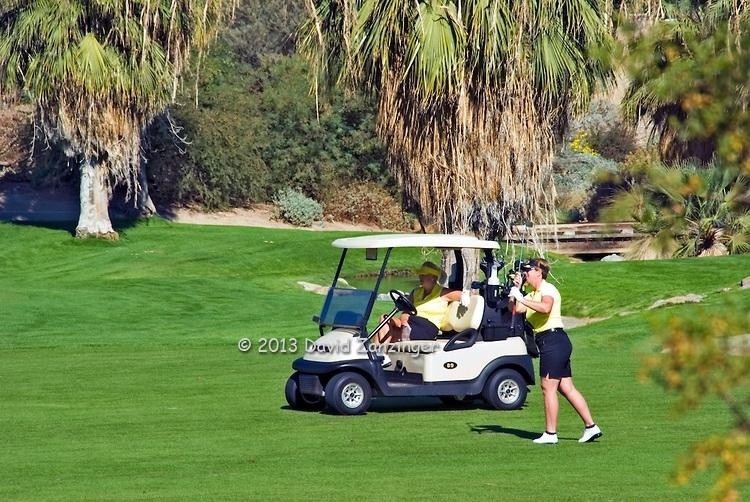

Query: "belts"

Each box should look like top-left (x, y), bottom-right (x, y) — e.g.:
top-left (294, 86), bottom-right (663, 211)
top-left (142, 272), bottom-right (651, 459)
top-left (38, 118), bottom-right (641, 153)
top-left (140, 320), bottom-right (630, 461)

top-left (536, 327), bottom-right (563, 337)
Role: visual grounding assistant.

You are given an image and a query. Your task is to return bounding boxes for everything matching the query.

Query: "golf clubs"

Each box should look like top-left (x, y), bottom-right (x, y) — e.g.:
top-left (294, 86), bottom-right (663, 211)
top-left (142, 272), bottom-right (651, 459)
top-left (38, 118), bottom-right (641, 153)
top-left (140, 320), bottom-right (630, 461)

top-left (510, 221), bottom-right (533, 331)
top-left (506, 259), bottom-right (529, 291)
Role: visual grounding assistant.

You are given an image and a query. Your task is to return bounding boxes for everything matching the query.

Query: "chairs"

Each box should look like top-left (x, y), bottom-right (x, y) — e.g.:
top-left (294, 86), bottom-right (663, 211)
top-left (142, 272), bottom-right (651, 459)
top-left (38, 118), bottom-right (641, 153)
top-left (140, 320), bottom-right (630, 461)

top-left (394, 294), bottom-right (485, 352)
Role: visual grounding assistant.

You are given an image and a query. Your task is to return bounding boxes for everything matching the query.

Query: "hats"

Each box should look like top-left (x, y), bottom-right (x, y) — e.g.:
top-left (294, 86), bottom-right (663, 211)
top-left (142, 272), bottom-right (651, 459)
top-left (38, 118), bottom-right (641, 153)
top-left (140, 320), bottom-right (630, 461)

top-left (415, 265), bottom-right (440, 277)
top-left (522, 257), bottom-right (549, 273)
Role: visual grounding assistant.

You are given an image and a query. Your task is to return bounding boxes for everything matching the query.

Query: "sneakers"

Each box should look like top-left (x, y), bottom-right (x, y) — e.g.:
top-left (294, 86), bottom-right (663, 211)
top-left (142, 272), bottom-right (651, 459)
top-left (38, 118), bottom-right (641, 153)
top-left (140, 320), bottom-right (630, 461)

top-left (578, 422), bottom-right (603, 443)
top-left (382, 353), bottom-right (391, 367)
top-left (533, 432), bottom-right (558, 444)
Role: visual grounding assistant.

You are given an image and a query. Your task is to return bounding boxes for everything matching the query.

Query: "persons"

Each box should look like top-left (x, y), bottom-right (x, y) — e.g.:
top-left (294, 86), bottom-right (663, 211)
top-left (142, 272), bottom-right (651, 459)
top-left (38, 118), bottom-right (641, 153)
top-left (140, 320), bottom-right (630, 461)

top-left (507, 256), bottom-right (604, 443)
top-left (370, 261), bottom-right (462, 345)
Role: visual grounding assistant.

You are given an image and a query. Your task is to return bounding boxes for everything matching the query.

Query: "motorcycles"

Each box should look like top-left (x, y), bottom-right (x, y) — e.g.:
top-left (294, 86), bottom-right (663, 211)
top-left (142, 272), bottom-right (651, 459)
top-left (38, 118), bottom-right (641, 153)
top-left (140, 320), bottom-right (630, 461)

top-left (282, 232), bottom-right (540, 417)
